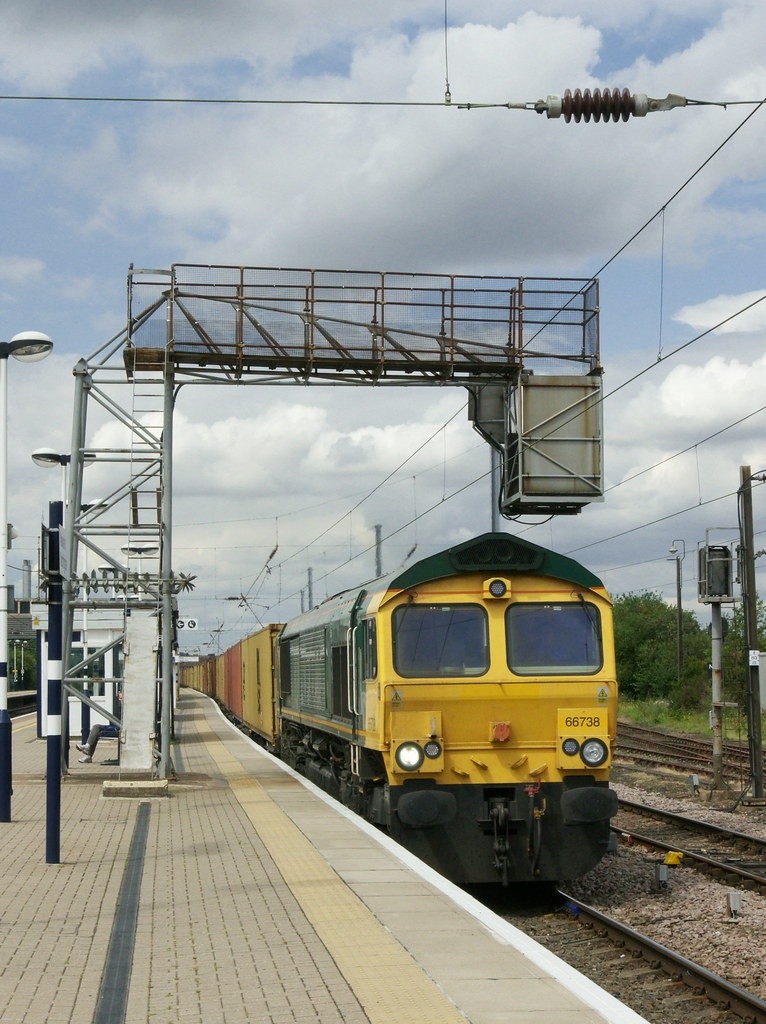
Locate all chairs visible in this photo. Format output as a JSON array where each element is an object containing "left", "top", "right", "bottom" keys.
[{"left": 99, "top": 737, "right": 121, "bottom": 765}]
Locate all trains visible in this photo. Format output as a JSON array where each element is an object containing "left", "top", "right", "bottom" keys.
[{"left": 179, "top": 532, "right": 618, "bottom": 886}]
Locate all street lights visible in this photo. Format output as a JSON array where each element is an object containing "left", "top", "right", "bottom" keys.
[
  {"left": 31, "top": 447, "right": 96, "bottom": 529},
  {"left": 61, "top": 499, "right": 110, "bottom": 745},
  {"left": 669, "top": 539, "right": 685, "bottom": 682},
  {"left": 0, "top": 330, "right": 54, "bottom": 822},
  {"left": 121, "top": 543, "right": 159, "bottom": 601}
]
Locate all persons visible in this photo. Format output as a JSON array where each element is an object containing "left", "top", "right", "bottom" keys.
[{"left": 76, "top": 692, "right": 122, "bottom": 763}]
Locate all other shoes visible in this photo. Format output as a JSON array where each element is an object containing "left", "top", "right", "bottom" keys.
[
  {"left": 76, "top": 744, "right": 90, "bottom": 755},
  {"left": 78, "top": 755, "right": 92, "bottom": 763}
]
